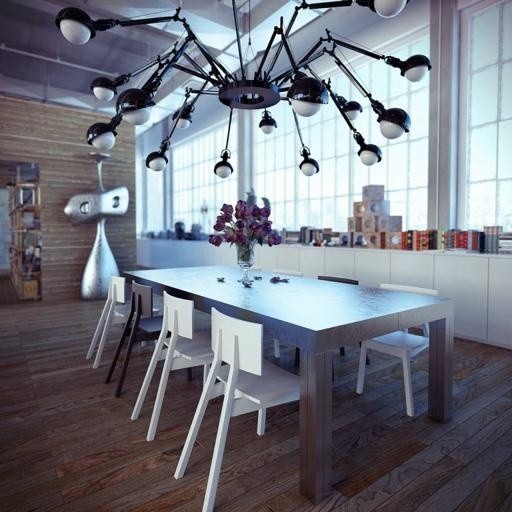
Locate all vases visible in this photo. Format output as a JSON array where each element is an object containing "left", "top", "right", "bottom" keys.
[{"left": 236, "top": 242, "right": 256, "bottom": 285}]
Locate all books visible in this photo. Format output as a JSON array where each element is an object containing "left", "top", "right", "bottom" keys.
[
  {"left": 299, "top": 227, "right": 332, "bottom": 245},
  {"left": 402, "top": 226, "right": 512, "bottom": 255}
]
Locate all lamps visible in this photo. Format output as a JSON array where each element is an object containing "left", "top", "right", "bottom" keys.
[{"left": 54, "top": 0, "right": 438, "bottom": 178}]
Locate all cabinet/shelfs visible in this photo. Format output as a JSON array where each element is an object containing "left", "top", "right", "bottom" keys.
[{"left": 6, "top": 182, "right": 42, "bottom": 304}]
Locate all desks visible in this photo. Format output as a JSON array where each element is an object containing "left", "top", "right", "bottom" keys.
[{"left": 123, "top": 266, "right": 454, "bottom": 506}]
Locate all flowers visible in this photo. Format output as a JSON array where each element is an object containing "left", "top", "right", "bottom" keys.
[{"left": 209, "top": 185, "right": 283, "bottom": 249}]
